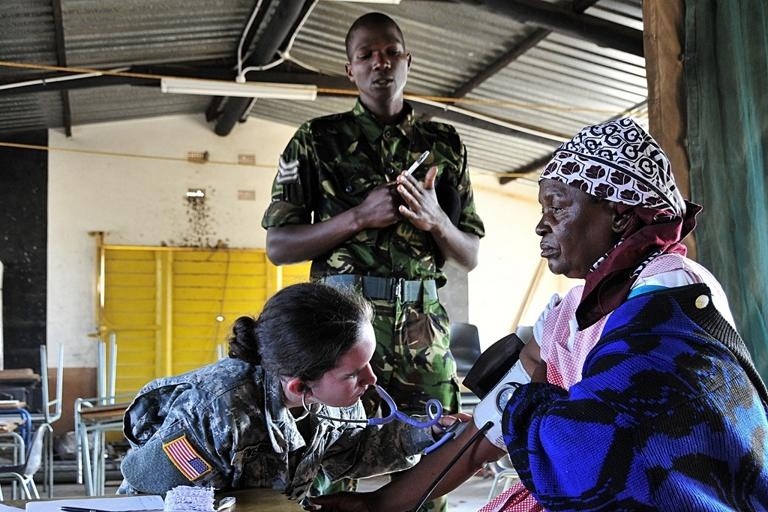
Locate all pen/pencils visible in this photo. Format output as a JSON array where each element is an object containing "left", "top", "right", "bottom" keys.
[
  {"left": 60, "top": 506, "right": 111, "bottom": 512},
  {"left": 408, "top": 151, "right": 429, "bottom": 176}
]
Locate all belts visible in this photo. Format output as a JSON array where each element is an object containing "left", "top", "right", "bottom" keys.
[{"left": 315, "top": 273, "right": 441, "bottom": 306}]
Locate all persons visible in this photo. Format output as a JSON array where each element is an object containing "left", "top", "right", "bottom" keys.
[
  {"left": 259, "top": 12, "right": 486, "bottom": 412},
  {"left": 115, "top": 281, "right": 472, "bottom": 504},
  {"left": 306, "top": 118, "right": 768, "bottom": 512}
]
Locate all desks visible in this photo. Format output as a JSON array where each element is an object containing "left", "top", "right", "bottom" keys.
[{"left": 0, "top": 486, "right": 305, "bottom": 511}]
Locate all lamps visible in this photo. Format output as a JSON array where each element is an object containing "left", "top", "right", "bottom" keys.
[{"left": 159, "top": 74, "right": 318, "bottom": 101}]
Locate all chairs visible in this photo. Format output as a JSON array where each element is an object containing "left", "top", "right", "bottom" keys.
[
  {"left": 449, "top": 322, "right": 482, "bottom": 407},
  {"left": 0, "top": 330, "right": 154, "bottom": 501}
]
